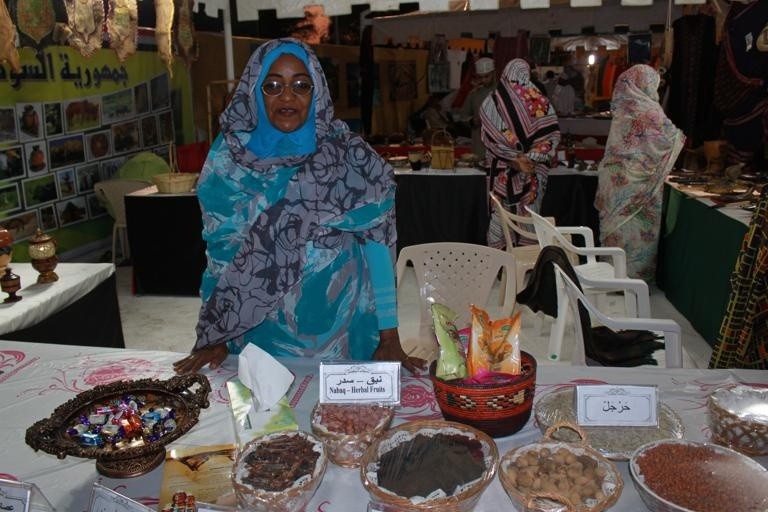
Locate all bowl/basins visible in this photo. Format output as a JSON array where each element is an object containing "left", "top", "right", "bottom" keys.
[
  {"left": 389, "top": 156, "right": 408, "bottom": 168},
  {"left": 629, "top": 436, "right": 768, "bottom": 511}
]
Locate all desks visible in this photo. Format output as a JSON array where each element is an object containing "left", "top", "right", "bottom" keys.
[
  {"left": 0, "top": 338, "right": 767, "bottom": 512},
  {"left": 127, "top": 178, "right": 207, "bottom": 297},
  {"left": 394, "top": 155, "right": 601, "bottom": 245},
  {"left": 656, "top": 171, "right": 768, "bottom": 349},
  {"left": 0, "top": 261, "right": 127, "bottom": 350}
]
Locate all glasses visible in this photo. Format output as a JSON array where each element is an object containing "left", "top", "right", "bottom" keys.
[{"left": 259, "top": 78, "right": 316, "bottom": 98}]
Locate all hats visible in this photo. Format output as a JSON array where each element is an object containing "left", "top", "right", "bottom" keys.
[
  {"left": 558, "top": 71, "right": 569, "bottom": 80},
  {"left": 474, "top": 57, "right": 497, "bottom": 75}
]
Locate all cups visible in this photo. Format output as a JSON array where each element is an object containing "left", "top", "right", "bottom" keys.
[{"left": 407, "top": 150, "right": 423, "bottom": 172}]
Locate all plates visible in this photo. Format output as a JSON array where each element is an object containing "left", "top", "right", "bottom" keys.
[{"left": 534, "top": 382, "right": 685, "bottom": 461}]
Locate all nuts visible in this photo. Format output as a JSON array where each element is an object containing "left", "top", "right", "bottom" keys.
[
  {"left": 632, "top": 443, "right": 768, "bottom": 512},
  {"left": 507, "top": 448, "right": 607, "bottom": 507}
]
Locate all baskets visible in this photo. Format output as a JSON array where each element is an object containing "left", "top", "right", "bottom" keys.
[
  {"left": 358, "top": 417, "right": 500, "bottom": 512},
  {"left": 230, "top": 425, "right": 331, "bottom": 512},
  {"left": 308, "top": 387, "right": 398, "bottom": 474},
  {"left": 497, "top": 420, "right": 627, "bottom": 512},
  {"left": 428, "top": 128, "right": 457, "bottom": 171},
  {"left": 707, "top": 380, "right": 768, "bottom": 457},
  {"left": 424, "top": 343, "right": 541, "bottom": 443},
  {"left": 149, "top": 139, "right": 201, "bottom": 195}
]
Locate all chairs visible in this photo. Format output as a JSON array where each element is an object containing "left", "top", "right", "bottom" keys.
[
  {"left": 484, "top": 192, "right": 578, "bottom": 305},
  {"left": 93, "top": 178, "right": 158, "bottom": 267},
  {"left": 394, "top": 241, "right": 518, "bottom": 361},
  {"left": 523, "top": 205, "right": 652, "bottom": 327},
  {"left": 547, "top": 262, "right": 683, "bottom": 367}
]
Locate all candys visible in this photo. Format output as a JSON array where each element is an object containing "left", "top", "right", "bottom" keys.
[{"left": 68, "top": 394, "right": 177, "bottom": 452}]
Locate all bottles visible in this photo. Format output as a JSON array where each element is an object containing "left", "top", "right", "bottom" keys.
[
  {"left": 28, "top": 228, "right": 59, "bottom": 283},
  {"left": 0, "top": 268, "right": 23, "bottom": 304},
  {"left": 567, "top": 143, "right": 576, "bottom": 169},
  {"left": 564, "top": 127, "right": 573, "bottom": 147}
]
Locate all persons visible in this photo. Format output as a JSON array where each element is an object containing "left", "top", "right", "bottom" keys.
[
  {"left": 532, "top": 65, "right": 597, "bottom": 118},
  {"left": 458, "top": 57, "right": 500, "bottom": 161},
  {"left": 171, "top": 38, "right": 398, "bottom": 379},
  {"left": 478, "top": 58, "right": 561, "bottom": 284},
  {"left": 593, "top": 63, "right": 687, "bottom": 297}
]
